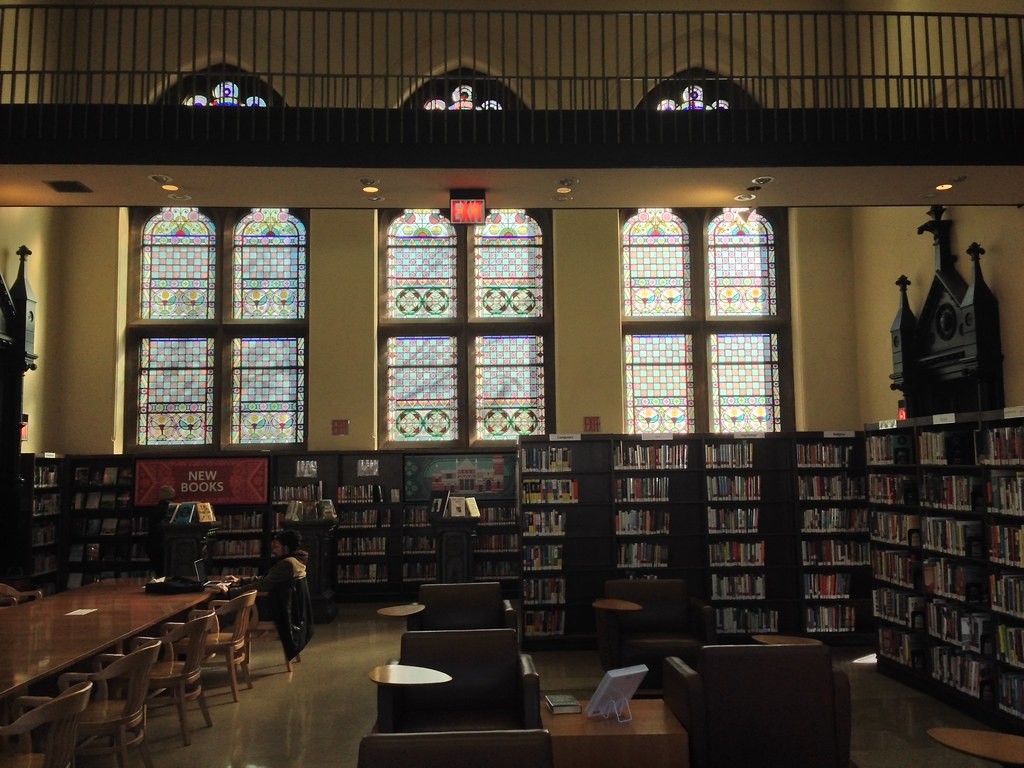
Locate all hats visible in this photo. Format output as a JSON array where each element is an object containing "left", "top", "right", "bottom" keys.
[{"left": 279, "top": 530, "right": 303, "bottom": 551}]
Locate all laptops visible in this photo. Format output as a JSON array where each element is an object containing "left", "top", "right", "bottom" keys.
[{"left": 194, "top": 558, "right": 232, "bottom": 586}]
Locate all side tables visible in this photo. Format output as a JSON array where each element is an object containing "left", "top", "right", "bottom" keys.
[{"left": 537, "top": 698, "right": 689, "bottom": 768}]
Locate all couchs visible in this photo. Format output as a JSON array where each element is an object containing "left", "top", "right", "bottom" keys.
[
  {"left": 357, "top": 728, "right": 554, "bottom": 768},
  {"left": 661, "top": 643, "right": 851, "bottom": 768},
  {"left": 591, "top": 579, "right": 716, "bottom": 692},
  {"left": 406, "top": 582, "right": 519, "bottom": 642},
  {"left": 374, "top": 628, "right": 542, "bottom": 733}
]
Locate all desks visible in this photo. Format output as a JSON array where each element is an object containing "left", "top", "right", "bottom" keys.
[{"left": 0, "top": 574, "right": 246, "bottom": 703}]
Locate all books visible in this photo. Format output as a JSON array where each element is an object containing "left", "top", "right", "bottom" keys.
[
  {"left": 522, "top": 446, "right": 581, "bottom": 636},
  {"left": 982, "top": 424, "right": 1023, "bottom": 722},
  {"left": 795, "top": 443, "right": 868, "bottom": 633},
  {"left": 401, "top": 489, "right": 524, "bottom": 583},
  {"left": 865, "top": 433, "right": 925, "bottom": 671},
  {"left": 611, "top": 440, "right": 691, "bottom": 579},
  {"left": 269, "top": 480, "right": 392, "bottom": 583},
  {"left": 33, "top": 462, "right": 267, "bottom": 598},
  {"left": 704, "top": 439, "right": 783, "bottom": 633},
  {"left": 916, "top": 432, "right": 996, "bottom": 701},
  {"left": 544, "top": 694, "right": 583, "bottom": 715}
]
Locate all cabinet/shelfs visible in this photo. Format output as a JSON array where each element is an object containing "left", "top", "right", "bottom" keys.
[{"left": 0, "top": 406, "right": 1024, "bottom": 740}]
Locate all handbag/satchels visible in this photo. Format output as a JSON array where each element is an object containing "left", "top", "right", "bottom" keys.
[{"left": 146, "top": 576, "right": 205, "bottom": 595}]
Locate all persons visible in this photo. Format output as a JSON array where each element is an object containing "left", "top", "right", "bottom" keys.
[
  {"left": 212, "top": 529, "right": 310, "bottom": 632},
  {"left": 146, "top": 486, "right": 176, "bottom": 578}
]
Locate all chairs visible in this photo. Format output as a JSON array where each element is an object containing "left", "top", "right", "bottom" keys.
[
  {"left": 0, "top": 680, "right": 93, "bottom": 768},
  {"left": 114, "top": 610, "right": 216, "bottom": 747},
  {"left": 15, "top": 639, "right": 162, "bottom": 768},
  {"left": 242, "top": 590, "right": 302, "bottom": 673},
  {"left": 162, "top": 589, "right": 259, "bottom": 703}
]
